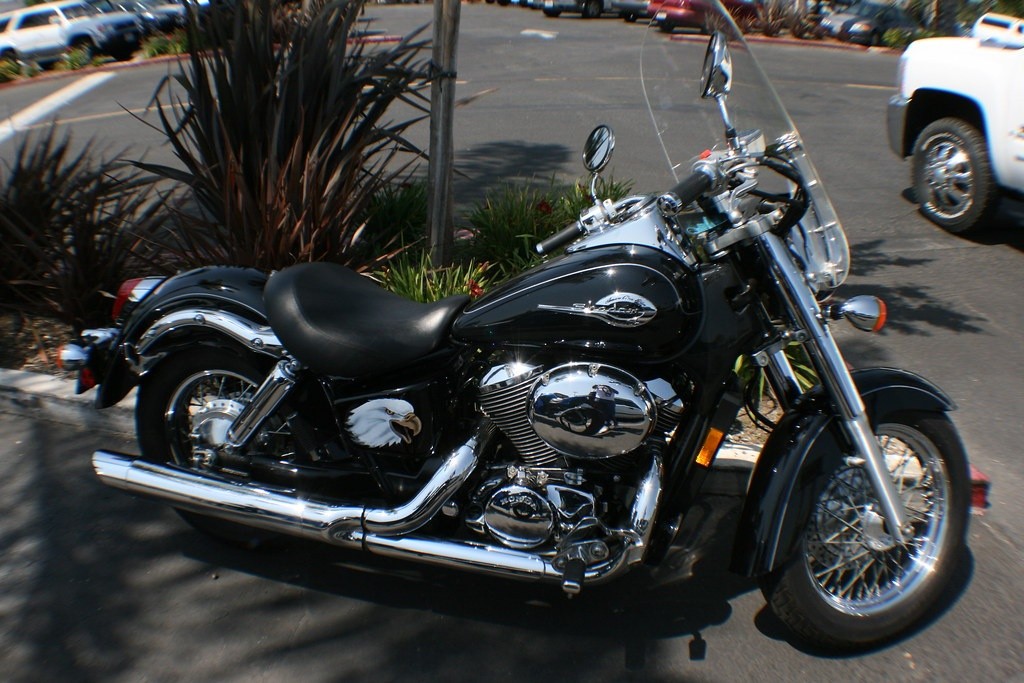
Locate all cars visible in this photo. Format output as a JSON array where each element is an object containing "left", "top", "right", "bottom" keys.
[
  {"left": 803, "top": 1, "right": 921, "bottom": 50},
  {"left": 534, "top": 0, "right": 769, "bottom": 38}
]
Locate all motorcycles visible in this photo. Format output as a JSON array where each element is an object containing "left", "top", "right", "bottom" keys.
[{"left": 50, "top": 0, "right": 973, "bottom": 650}]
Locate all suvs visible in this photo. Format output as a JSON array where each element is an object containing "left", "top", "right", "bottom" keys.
[
  {"left": 0, "top": 0, "right": 212, "bottom": 66},
  {"left": 886, "top": 13, "right": 1023, "bottom": 239}
]
